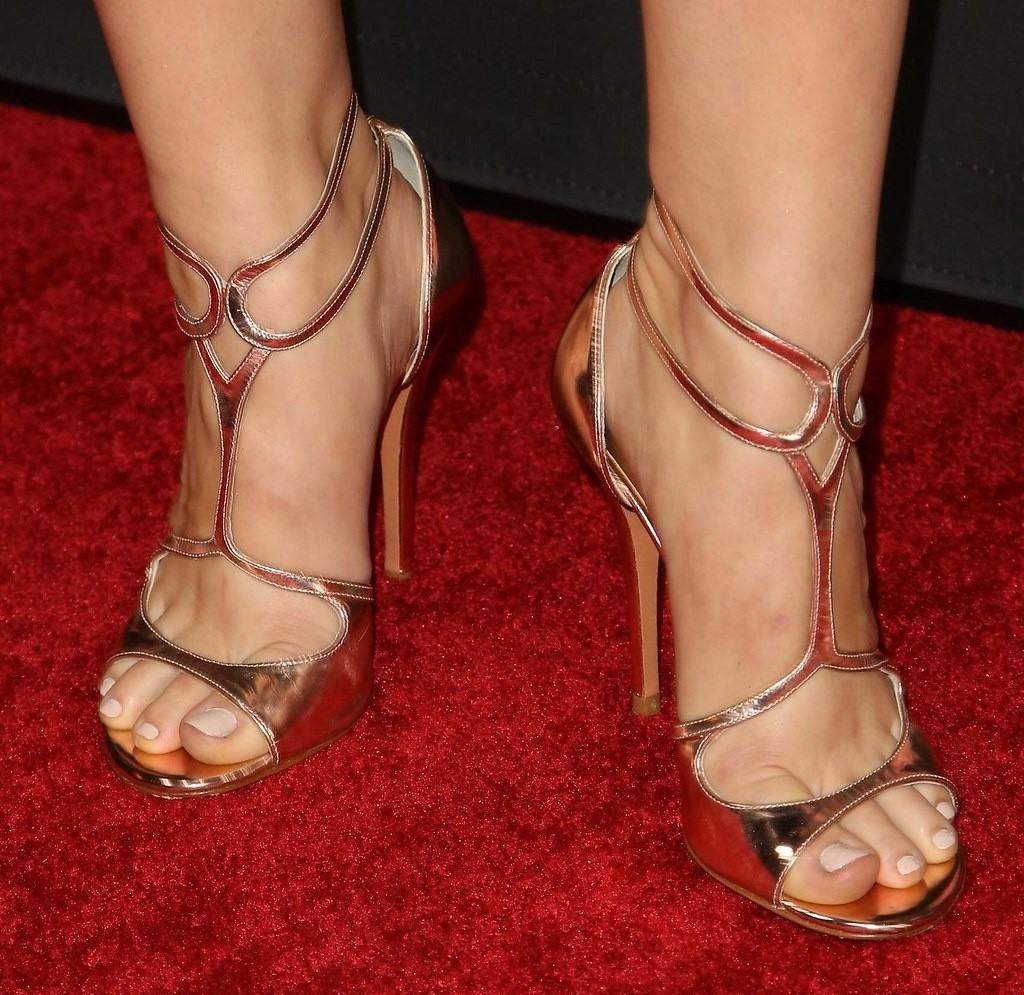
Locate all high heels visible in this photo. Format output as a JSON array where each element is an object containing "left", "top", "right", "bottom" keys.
[
  {"left": 99, "top": 87, "right": 491, "bottom": 801},
  {"left": 549, "top": 191, "right": 968, "bottom": 940}
]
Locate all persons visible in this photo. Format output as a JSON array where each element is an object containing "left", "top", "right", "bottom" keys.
[{"left": 96, "top": 0, "right": 964, "bottom": 942}]
